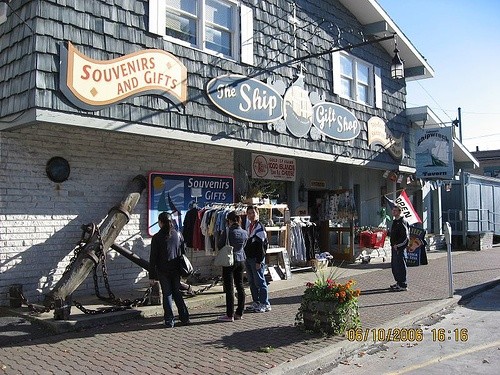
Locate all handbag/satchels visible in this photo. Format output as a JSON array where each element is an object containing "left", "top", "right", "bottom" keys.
[
  {"left": 213, "top": 245, "right": 234, "bottom": 266},
  {"left": 180, "top": 253, "right": 194, "bottom": 277}
]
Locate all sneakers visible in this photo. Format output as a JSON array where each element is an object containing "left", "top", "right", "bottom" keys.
[
  {"left": 246, "top": 300, "right": 261, "bottom": 312},
  {"left": 254, "top": 301, "right": 271, "bottom": 312},
  {"left": 218, "top": 314, "right": 235, "bottom": 321},
  {"left": 234, "top": 312, "right": 242, "bottom": 320}
]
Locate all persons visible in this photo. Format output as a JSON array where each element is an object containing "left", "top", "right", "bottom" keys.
[
  {"left": 390, "top": 206, "right": 410, "bottom": 291},
  {"left": 149, "top": 212, "right": 191, "bottom": 328},
  {"left": 217, "top": 211, "right": 248, "bottom": 321},
  {"left": 244, "top": 205, "right": 271, "bottom": 312}
]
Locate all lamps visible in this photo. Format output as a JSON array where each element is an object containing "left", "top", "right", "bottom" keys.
[{"left": 314, "top": 32, "right": 404, "bottom": 82}]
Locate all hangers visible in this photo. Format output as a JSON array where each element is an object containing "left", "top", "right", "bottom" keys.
[
  {"left": 193, "top": 203, "right": 246, "bottom": 209},
  {"left": 290, "top": 216, "right": 310, "bottom": 224}
]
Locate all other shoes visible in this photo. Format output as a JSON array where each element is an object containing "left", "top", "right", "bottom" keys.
[
  {"left": 390, "top": 282, "right": 407, "bottom": 291},
  {"left": 182, "top": 319, "right": 191, "bottom": 326},
  {"left": 165, "top": 320, "right": 175, "bottom": 328}
]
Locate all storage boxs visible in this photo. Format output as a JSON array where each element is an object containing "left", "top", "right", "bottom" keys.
[
  {"left": 359, "top": 232, "right": 385, "bottom": 248},
  {"left": 243, "top": 198, "right": 260, "bottom": 204}
]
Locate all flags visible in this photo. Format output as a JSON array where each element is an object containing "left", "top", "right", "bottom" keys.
[
  {"left": 384, "top": 190, "right": 422, "bottom": 226},
  {"left": 382, "top": 170, "right": 450, "bottom": 191}
]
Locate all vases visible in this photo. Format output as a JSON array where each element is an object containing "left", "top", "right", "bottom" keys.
[{"left": 304, "top": 299, "right": 352, "bottom": 334}]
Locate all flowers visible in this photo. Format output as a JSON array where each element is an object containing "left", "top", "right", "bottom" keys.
[{"left": 294, "top": 259, "right": 362, "bottom": 334}]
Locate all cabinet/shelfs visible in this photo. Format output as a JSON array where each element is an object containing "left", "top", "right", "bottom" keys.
[{"left": 243, "top": 203, "right": 290, "bottom": 268}]
